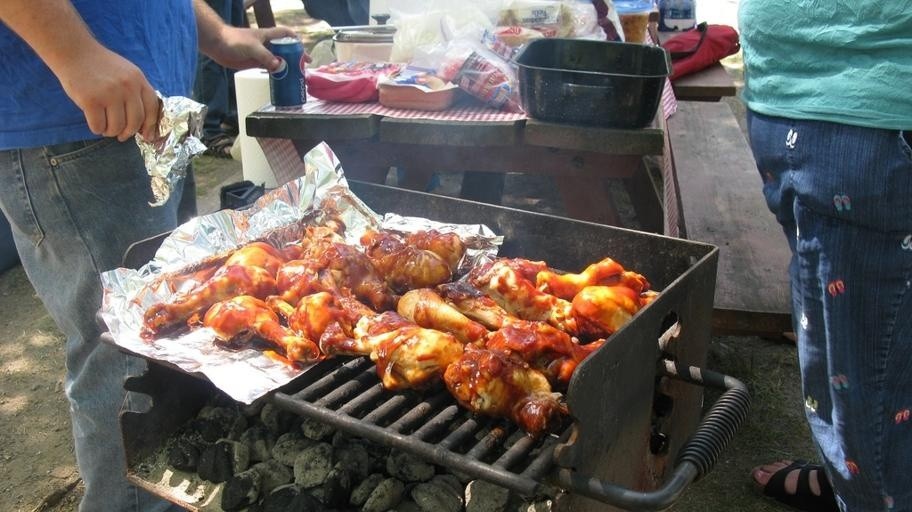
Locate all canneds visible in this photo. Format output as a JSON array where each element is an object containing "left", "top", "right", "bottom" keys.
[{"left": 268, "top": 35, "right": 306, "bottom": 107}]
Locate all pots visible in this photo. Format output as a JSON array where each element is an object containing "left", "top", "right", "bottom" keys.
[{"left": 334, "top": 14, "right": 396, "bottom": 63}]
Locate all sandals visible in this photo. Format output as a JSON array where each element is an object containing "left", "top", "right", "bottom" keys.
[
  {"left": 199, "top": 137, "right": 232, "bottom": 157},
  {"left": 748, "top": 458, "right": 838, "bottom": 509}
]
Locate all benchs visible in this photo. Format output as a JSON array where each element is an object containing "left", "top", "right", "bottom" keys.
[
  {"left": 647, "top": 22, "right": 737, "bottom": 102},
  {"left": 666, "top": 103, "right": 796, "bottom": 341}
]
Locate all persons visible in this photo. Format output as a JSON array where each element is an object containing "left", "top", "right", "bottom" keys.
[
  {"left": 729, "top": 0, "right": 910, "bottom": 509},
  {"left": 1, "top": 0, "right": 315, "bottom": 512},
  {"left": 197, "top": 0, "right": 256, "bottom": 163}
]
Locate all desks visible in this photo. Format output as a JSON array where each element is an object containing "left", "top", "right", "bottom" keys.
[{"left": 246, "top": 93, "right": 664, "bottom": 227}]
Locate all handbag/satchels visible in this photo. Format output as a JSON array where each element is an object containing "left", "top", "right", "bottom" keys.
[{"left": 663, "top": 20, "right": 743, "bottom": 84}]
